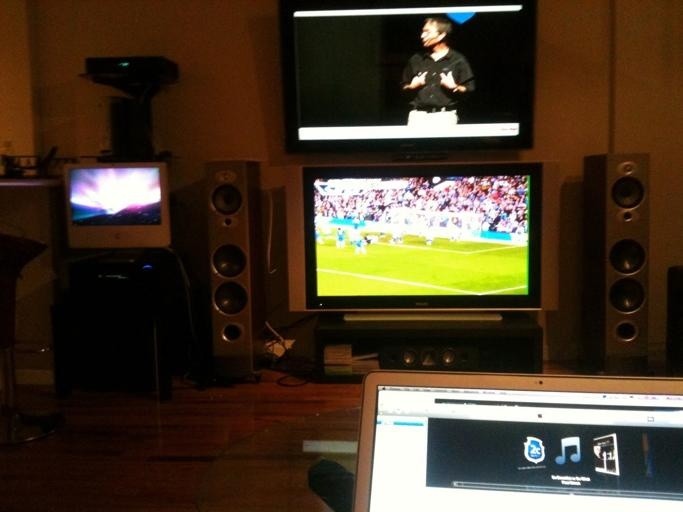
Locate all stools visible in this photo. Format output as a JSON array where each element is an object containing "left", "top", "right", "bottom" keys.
[{"left": 0, "top": 233, "right": 63, "bottom": 446}]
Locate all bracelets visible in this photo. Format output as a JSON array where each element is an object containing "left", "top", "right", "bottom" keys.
[{"left": 452, "top": 85, "right": 461, "bottom": 93}]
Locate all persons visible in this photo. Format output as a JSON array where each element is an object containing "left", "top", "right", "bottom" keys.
[
  {"left": 400, "top": 17, "right": 476, "bottom": 125},
  {"left": 313, "top": 175, "right": 529, "bottom": 257}
]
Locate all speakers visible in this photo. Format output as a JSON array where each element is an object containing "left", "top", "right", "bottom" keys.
[
  {"left": 581, "top": 154, "right": 649, "bottom": 375},
  {"left": 208, "top": 161, "right": 263, "bottom": 383},
  {"left": 395, "top": 343, "right": 462, "bottom": 370}
]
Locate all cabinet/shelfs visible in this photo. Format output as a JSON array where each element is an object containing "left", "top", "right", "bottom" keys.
[{"left": 314, "top": 312, "right": 543, "bottom": 383}]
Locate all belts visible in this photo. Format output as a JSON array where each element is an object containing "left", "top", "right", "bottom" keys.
[{"left": 416, "top": 106, "right": 457, "bottom": 113}]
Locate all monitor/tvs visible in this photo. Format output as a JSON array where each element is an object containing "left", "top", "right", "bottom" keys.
[
  {"left": 284, "top": 161, "right": 561, "bottom": 312},
  {"left": 279, "top": 0, "right": 537, "bottom": 154},
  {"left": 352, "top": 370, "right": 683, "bottom": 512},
  {"left": 64, "top": 162, "right": 171, "bottom": 263}
]
